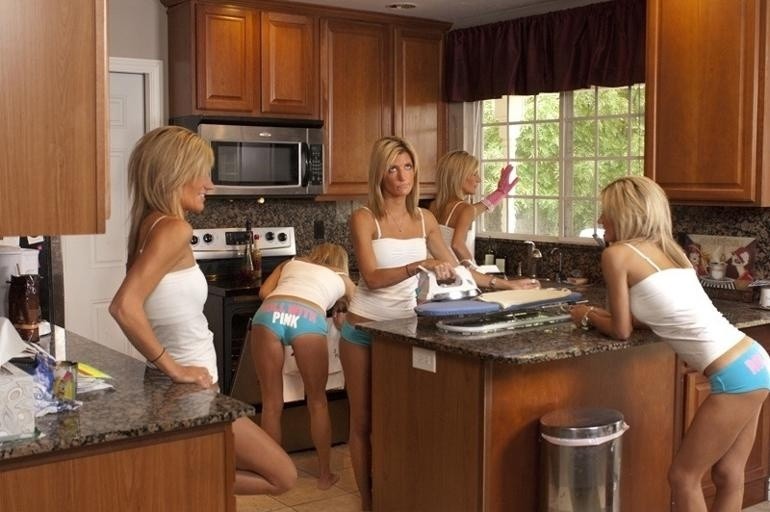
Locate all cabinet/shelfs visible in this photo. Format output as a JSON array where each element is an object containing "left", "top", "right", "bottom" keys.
[
  {"left": 315, "top": 6, "right": 453, "bottom": 203},
  {"left": 643, "top": 0, "right": 769, "bottom": 209},
  {"left": 162, "top": 0, "right": 323, "bottom": 127},
  {"left": 1, "top": 323, "right": 256, "bottom": 511},
  {"left": 354, "top": 302, "right": 769, "bottom": 510},
  {"left": 0, "top": 0, "right": 112, "bottom": 235}
]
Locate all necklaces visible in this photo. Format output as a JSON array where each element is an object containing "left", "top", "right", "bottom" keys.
[{"left": 385, "top": 209, "right": 407, "bottom": 232}]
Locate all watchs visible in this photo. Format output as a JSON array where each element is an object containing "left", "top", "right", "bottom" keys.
[{"left": 580, "top": 307, "right": 594, "bottom": 331}]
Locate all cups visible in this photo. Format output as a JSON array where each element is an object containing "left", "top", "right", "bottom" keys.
[{"left": 704, "top": 262, "right": 728, "bottom": 280}]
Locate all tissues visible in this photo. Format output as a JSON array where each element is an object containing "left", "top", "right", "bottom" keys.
[{"left": 0, "top": 317, "right": 36, "bottom": 443}]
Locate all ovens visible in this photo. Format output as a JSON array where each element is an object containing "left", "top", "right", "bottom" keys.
[{"left": 198, "top": 124, "right": 324, "bottom": 196}]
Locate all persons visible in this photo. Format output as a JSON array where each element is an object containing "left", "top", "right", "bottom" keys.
[
  {"left": 570, "top": 175, "right": 766, "bottom": 512},
  {"left": 338, "top": 137, "right": 539, "bottom": 512},
  {"left": 249, "top": 244, "right": 357, "bottom": 490},
  {"left": 429, "top": 151, "right": 521, "bottom": 271},
  {"left": 110, "top": 126, "right": 297, "bottom": 495}
]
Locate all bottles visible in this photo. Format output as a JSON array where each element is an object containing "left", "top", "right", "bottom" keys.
[
  {"left": 494, "top": 248, "right": 508, "bottom": 275},
  {"left": 483, "top": 244, "right": 495, "bottom": 266}
]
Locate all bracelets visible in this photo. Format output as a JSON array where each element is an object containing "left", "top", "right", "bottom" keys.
[
  {"left": 406, "top": 265, "right": 411, "bottom": 278},
  {"left": 147, "top": 348, "right": 165, "bottom": 364},
  {"left": 489, "top": 277, "right": 497, "bottom": 289}
]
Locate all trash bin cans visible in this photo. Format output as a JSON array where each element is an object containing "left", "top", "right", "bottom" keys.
[{"left": 539, "top": 404, "right": 626, "bottom": 512}]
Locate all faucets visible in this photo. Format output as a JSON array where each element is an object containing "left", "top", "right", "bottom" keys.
[
  {"left": 549, "top": 247, "right": 565, "bottom": 283},
  {"left": 522, "top": 240, "right": 543, "bottom": 278}
]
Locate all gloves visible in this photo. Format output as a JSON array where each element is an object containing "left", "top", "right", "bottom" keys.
[{"left": 478, "top": 164, "right": 521, "bottom": 211}]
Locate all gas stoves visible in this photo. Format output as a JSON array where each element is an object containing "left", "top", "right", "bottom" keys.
[{"left": 205, "top": 267, "right": 264, "bottom": 292}]
